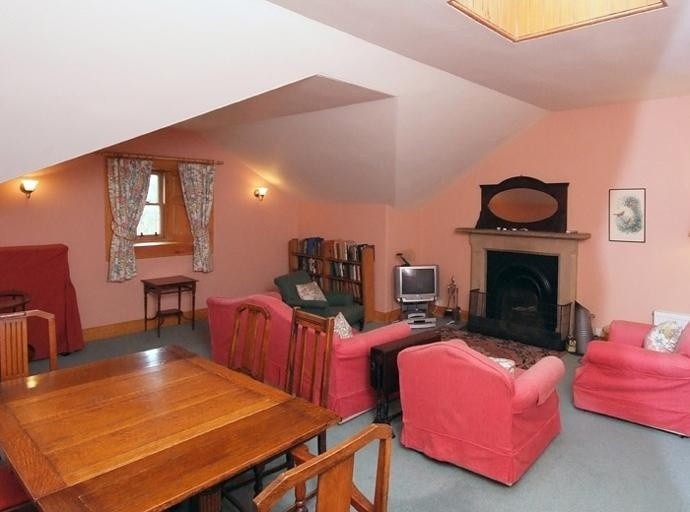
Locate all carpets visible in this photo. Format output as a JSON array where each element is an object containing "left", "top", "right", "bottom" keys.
[{"left": 433, "top": 326, "right": 567, "bottom": 372}]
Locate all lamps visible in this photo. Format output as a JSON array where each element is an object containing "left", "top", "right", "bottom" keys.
[
  {"left": 20, "top": 179, "right": 40, "bottom": 199},
  {"left": 254, "top": 187, "right": 269, "bottom": 201}
]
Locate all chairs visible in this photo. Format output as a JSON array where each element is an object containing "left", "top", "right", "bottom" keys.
[
  {"left": 251, "top": 424, "right": 395, "bottom": 512},
  {"left": 228, "top": 301, "right": 271, "bottom": 383},
  {"left": 0, "top": 309, "right": 58, "bottom": 384},
  {"left": 224, "top": 308, "right": 335, "bottom": 512}
]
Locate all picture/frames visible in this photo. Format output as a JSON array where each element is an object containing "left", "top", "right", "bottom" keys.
[{"left": 608, "top": 187, "right": 646, "bottom": 244}]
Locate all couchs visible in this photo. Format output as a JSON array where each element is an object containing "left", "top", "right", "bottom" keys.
[
  {"left": 571, "top": 318, "right": 690, "bottom": 439},
  {"left": 207, "top": 270, "right": 413, "bottom": 422},
  {"left": 398, "top": 338, "right": 567, "bottom": 487}
]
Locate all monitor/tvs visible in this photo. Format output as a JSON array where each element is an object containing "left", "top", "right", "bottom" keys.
[{"left": 395, "top": 266, "right": 438, "bottom": 300}]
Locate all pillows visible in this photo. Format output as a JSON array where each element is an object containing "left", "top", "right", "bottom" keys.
[
  {"left": 493, "top": 356, "right": 515, "bottom": 374},
  {"left": 642, "top": 321, "right": 682, "bottom": 355},
  {"left": 334, "top": 311, "right": 354, "bottom": 339},
  {"left": 297, "top": 282, "right": 328, "bottom": 303},
  {"left": 274, "top": 269, "right": 329, "bottom": 309}
]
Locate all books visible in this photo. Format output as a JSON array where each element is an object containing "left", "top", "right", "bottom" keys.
[
  {"left": 332, "top": 278, "right": 361, "bottom": 299},
  {"left": 296, "top": 256, "right": 322, "bottom": 274},
  {"left": 329, "top": 241, "right": 368, "bottom": 262},
  {"left": 296, "top": 236, "right": 324, "bottom": 257},
  {"left": 313, "top": 275, "right": 323, "bottom": 289},
  {"left": 331, "top": 261, "right": 362, "bottom": 281}
]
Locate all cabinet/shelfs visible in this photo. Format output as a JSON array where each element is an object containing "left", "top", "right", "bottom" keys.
[{"left": 288, "top": 238, "right": 375, "bottom": 322}]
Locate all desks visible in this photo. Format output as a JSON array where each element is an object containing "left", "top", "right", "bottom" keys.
[
  {"left": 0, "top": 341, "right": 343, "bottom": 512},
  {"left": 140, "top": 276, "right": 200, "bottom": 337}
]
